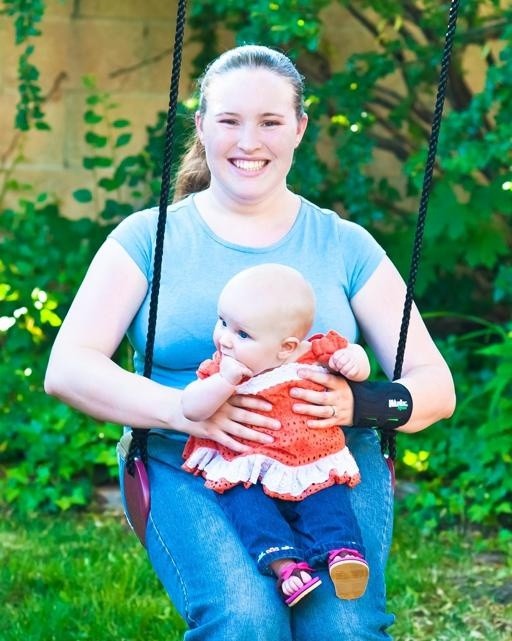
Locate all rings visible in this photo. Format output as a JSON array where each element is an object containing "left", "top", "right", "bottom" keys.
[{"left": 330, "top": 406, "right": 336, "bottom": 417}]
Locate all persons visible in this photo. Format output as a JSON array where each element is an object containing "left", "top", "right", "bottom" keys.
[
  {"left": 42, "top": 42, "right": 457, "bottom": 641},
  {"left": 180, "top": 262, "right": 371, "bottom": 609}
]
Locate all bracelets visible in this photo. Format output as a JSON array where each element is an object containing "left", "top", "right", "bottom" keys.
[{"left": 346, "top": 378, "right": 413, "bottom": 432}]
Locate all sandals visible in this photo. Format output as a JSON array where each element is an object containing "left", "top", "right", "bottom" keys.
[
  {"left": 328, "top": 548, "right": 369, "bottom": 600},
  {"left": 278, "top": 562, "right": 322, "bottom": 608}
]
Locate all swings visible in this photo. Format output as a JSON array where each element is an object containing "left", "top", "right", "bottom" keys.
[{"left": 123, "top": 0, "right": 460, "bottom": 544}]
